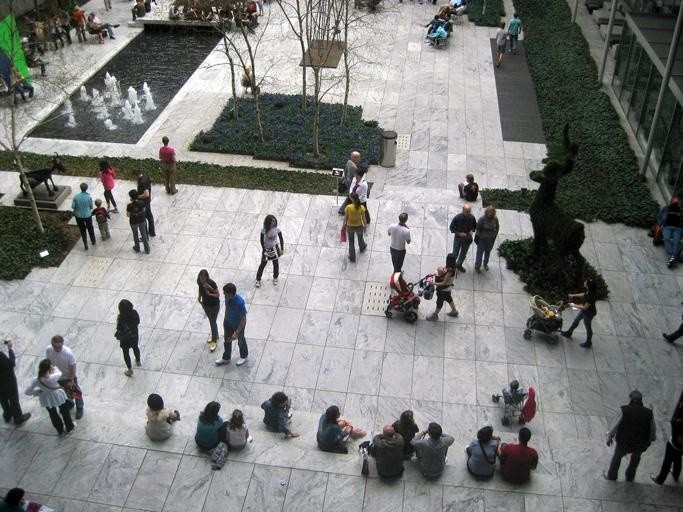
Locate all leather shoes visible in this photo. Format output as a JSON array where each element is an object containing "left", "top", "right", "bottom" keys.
[{"left": 13, "top": 413, "right": 31, "bottom": 424}]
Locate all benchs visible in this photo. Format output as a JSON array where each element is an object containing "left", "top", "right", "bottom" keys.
[
  {"left": 431, "top": 3, "right": 463, "bottom": 50},
  {"left": 0, "top": 8, "right": 110, "bottom": 100}
]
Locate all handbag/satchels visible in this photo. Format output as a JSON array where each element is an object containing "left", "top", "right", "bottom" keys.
[
  {"left": 209, "top": 443, "right": 228, "bottom": 471},
  {"left": 65, "top": 398, "right": 74, "bottom": 410},
  {"left": 341, "top": 226, "right": 347, "bottom": 242},
  {"left": 425, "top": 276, "right": 436, "bottom": 299},
  {"left": 121, "top": 323, "right": 135, "bottom": 344},
  {"left": 264, "top": 244, "right": 282, "bottom": 261},
  {"left": 339, "top": 178, "right": 347, "bottom": 193},
  {"left": 360, "top": 445, "right": 371, "bottom": 475}
]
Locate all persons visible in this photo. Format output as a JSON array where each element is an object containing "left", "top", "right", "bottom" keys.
[
  {"left": 386, "top": 212, "right": 411, "bottom": 274},
  {"left": 260, "top": 392, "right": 300, "bottom": 437},
  {"left": 196, "top": 269, "right": 219, "bottom": 353},
  {"left": 256, "top": 214, "right": 284, "bottom": 289},
  {"left": 506, "top": 12, "right": 522, "bottom": 57},
  {"left": 338, "top": 151, "right": 360, "bottom": 214},
  {"left": 656, "top": 196, "right": 682, "bottom": 267},
  {"left": 24, "top": 358, "right": 76, "bottom": 434},
  {"left": 342, "top": 193, "right": 368, "bottom": 264},
  {"left": 370, "top": 410, "right": 419, "bottom": 483},
  {"left": 466, "top": 426, "right": 500, "bottom": 481},
  {"left": 421, "top": 0, "right": 468, "bottom": 48},
  {"left": 475, "top": 206, "right": 499, "bottom": 274},
  {"left": 409, "top": 423, "right": 455, "bottom": 479},
  {"left": 90, "top": 199, "right": 110, "bottom": 241},
  {"left": 494, "top": 22, "right": 508, "bottom": 68},
  {"left": 662, "top": 321, "right": 682, "bottom": 345},
  {"left": 45, "top": 334, "right": 82, "bottom": 420},
  {"left": 131, "top": 168, "right": 154, "bottom": 237},
  {"left": 448, "top": 205, "right": 477, "bottom": 272},
  {"left": 0, "top": 1, "right": 264, "bottom": 103},
  {"left": 98, "top": 159, "right": 119, "bottom": 214},
  {"left": 158, "top": 136, "right": 178, "bottom": 194},
  {"left": 126, "top": 188, "right": 149, "bottom": 255},
  {"left": 457, "top": 173, "right": 478, "bottom": 202},
  {"left": 112, "top": 298, "right": 142, "bottom": 378},
  {"left": 213, "top": 283, "right": 250, "bottom": 367},
  {"left": 70, "top": 183, "right": 95, "bottom": 250},
  {"left": 650, "top": 395, "right": 683, "bottom": 483},
  {"left": 0, "top": 486, "right": 53, "bottom": 511},
  {"left": 195, "top": 401, "right": 250, "bottom": 451},
  {"left": 0, "top": 336, "right": 30, "bottom": 425},
  {"left": 316, "top": 406, "right": 366, "bottom": 454},
  {"left": 349, "top": 167, "right": 370, "bottom": 224},
  {"left": 604, "top": 390, "right": 655, "bottom": 483},
  {"left": 561, "top": 275, "right": 597, "bottom": 349},
  {"left": 425, "top": 253, "right": 461, "bottom": 321},
  {"left": 498, "top": 428, "right": 537, "bottom": 485},
  {"left": 143, "top": 393, "right": 179, "bottom": 443},
  {"left": 503, "top": 380, "right": 525, "bottom": 404}
]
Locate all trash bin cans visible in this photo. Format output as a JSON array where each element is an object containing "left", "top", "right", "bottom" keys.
[{"left": 380, "top": 131, "right": 398, "bottom": 167}]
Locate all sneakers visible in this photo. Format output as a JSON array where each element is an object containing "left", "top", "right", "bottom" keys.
[
  {"left": 425, "top": 312, "right": 438, "bottom": 320},
  {"left": 650, "top": 473, "right": 657, "bottom": 480},
  {"left": 447, "top": 311, "right": 458, "bottom": 315},
  {"left": 236, "top": 358, "right": 248, "bottom": 365},
  {"left": 209, "top": 342, "right": 217, "bottom": 351},
  {"left": 273, "top": 278, "right": 278, "bottom": 285},
  {"left": 76, "top": 412, "right": 82, "bottom": 419},
  {"left": 215, "top": 358, "right": 231, "bottom": 364},
  {"left": 255, "top": 281, "right": 260, "bottom": 287},
  {"left": 66, "top": 421, "right": 77, "bottom": 433}
]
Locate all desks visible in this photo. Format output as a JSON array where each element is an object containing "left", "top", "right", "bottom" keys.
[{"left": 585, "top": 0, "right": 643, "bottom": 61}]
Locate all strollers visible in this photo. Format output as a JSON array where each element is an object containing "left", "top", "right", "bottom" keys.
[
  {"left": 383, "top": 271, "right": 436, "bottom": 324},
  {"left": 523, "top": 293, "right": 570, "bottom": 345},
  {"left": 492, "top": 380, "right": 536, "bottom": 427}
]
